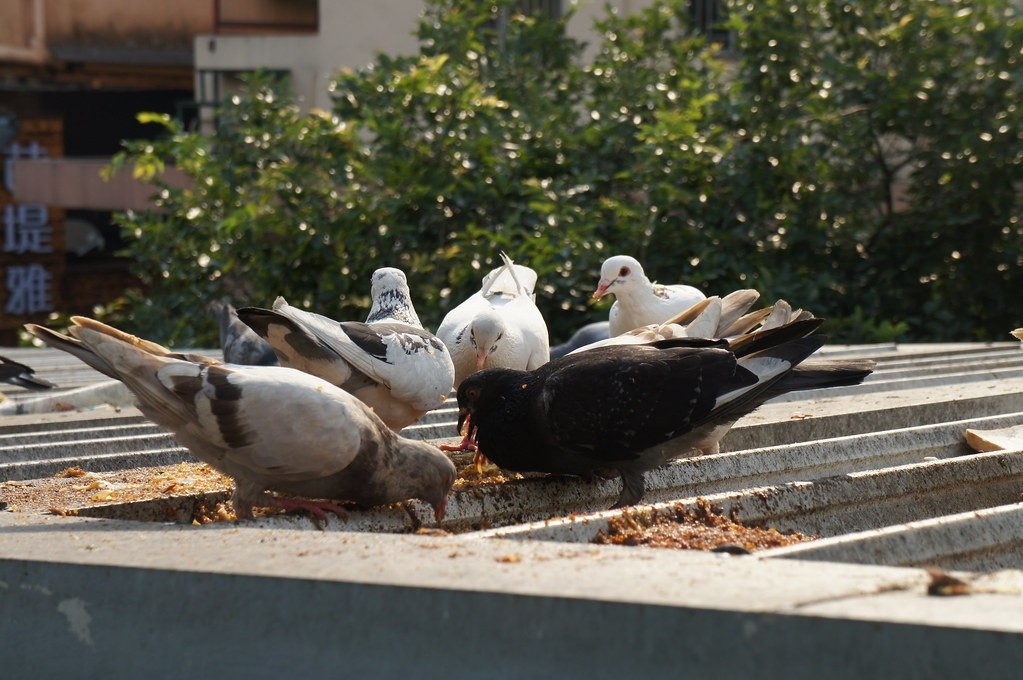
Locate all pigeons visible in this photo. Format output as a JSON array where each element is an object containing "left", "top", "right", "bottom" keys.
[{"left": 22, "top": 251, "right": 876, "bottom": 529}]
